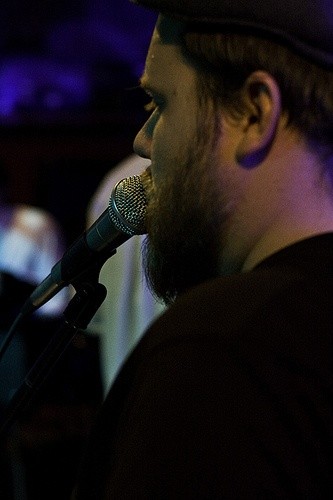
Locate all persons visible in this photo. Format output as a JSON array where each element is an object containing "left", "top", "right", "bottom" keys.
[
  {"left": 47, "top": 0, "right": 332, "bottom": 498},
  {"left": 0, "top": 47, "right": 178, "bottom": 402}
]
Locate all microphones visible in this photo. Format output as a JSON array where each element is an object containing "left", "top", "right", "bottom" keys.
[{"left": 27, "top": 174, "right": 149, "bottom": 314}]
